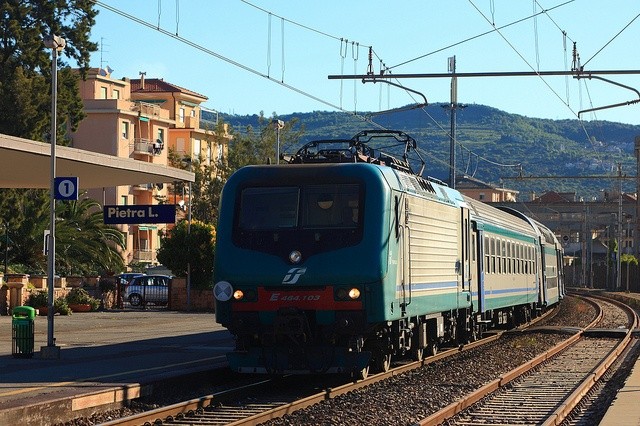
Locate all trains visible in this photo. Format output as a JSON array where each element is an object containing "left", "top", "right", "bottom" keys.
[{"left": 213, "top": 130, "right": 564, "bottom": 380}]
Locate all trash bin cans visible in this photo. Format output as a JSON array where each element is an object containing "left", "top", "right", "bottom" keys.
[{"left": 12, "top": 306, "right": 35, "bottom": 353}]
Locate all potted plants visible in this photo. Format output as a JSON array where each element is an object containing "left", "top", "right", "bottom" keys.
[
  {"left": 28, "top": 290, "right": 74, "bottom": 316},
  {"left": 64, "top": 287, "right": 101, "bottom": 312}
]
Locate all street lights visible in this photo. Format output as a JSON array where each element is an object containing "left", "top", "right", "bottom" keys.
[
  {"left": 41, "top": 35, "right": 66, "bottom": 359},
  {"left": 272, "top": 119, "right": 285, "bottom": 165}
]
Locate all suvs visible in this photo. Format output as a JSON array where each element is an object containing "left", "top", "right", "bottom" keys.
[
  {"left": 119, "top": 273, "right": 167, "bottom": 306},
  {"left": 124, "top": 275, "right": 170, "bottom": 307}
]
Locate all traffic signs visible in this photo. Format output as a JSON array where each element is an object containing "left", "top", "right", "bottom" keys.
[{"left": 55, "top": 177, "right": 77, "bottom": 199}]
